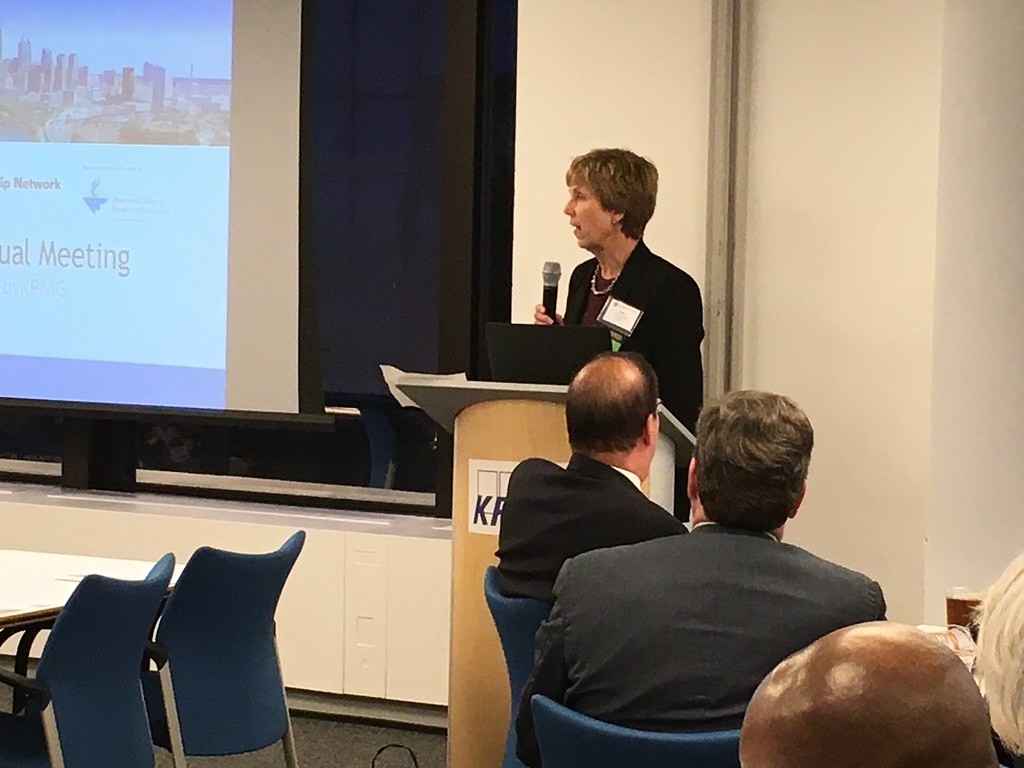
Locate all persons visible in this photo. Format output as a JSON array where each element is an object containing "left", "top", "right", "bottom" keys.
[
  {"left": 976, "top": 553, "right": 1024, "bottom": 768},
  {"left": 536, "top": 148, "right": 703, "bottom": 521},
  {"left": 512, "top": 390, "right": 887, "bottom": 768},
  {"left": 739, "top": 621, "right": 995, "bottom": 767},
  {"left": 494, "top": 352, "right": 689, "bottom": 601}
]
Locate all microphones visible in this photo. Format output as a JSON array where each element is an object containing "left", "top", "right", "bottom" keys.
[{"left": 543, "top": 262, "right": 562, "bottom": 324}]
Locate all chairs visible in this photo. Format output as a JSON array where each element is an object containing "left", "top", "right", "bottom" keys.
[
  {"left": 0, "top": 529, "right": 308, "bottom": 768},
  {"left": 484, "top": 566, "right": 741, "bottom": 768}
]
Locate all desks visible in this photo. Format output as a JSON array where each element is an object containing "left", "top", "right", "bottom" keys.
[{"left": 0, "top": 548, "right": 186, "bottom": 714}]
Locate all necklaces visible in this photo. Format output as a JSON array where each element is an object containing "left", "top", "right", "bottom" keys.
[{"left": 591, "top": 260, "right": 624, "bottom": 296}]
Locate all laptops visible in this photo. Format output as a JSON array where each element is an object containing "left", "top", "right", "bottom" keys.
[{"left": 486, "top": 322, "right": 613, "bottom": 385}]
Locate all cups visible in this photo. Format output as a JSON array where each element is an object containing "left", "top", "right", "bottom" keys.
[{"left": 943, "top": 587, "right": 982, "bottom": 644}]
